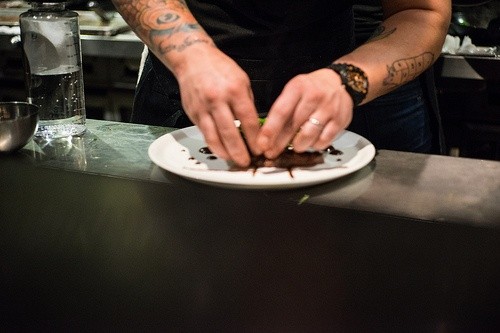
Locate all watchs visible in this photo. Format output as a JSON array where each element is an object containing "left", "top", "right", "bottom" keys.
[{"left": 325, "top": 63, "right": 370, "bottom": 106}]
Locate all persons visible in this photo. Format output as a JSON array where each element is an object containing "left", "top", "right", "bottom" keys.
[{"left": 111, "top": 0, "right": 452, "bottom": 168}]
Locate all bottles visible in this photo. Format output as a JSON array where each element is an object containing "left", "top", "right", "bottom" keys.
[{"left": 19, "top": 0, "right": 87, "bottom": 139}]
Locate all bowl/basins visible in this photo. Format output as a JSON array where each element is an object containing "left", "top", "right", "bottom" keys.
[{"left": 0, "top": 102, "right": 39, "bottom": 155}]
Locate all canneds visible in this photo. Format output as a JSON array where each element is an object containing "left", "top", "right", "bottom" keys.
[{"left": 451, "top": 12, "right": 470, "bottom": 32}]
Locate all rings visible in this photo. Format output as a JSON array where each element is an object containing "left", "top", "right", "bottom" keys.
[{"left": 308, "top": 118, "right": 324, "bottom": 130}]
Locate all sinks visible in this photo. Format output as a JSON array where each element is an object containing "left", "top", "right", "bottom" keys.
[{"left": 0, "top": 8, "right": 131, "bottom": 37}]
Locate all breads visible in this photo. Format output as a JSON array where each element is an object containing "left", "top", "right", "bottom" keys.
[{"left": 243, "top": 148, "right": 323, "bottom": 166}]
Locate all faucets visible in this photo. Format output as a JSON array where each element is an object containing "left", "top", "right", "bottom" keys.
[{"left": 89, "top": 1, "right": 110, "bottom": 23}]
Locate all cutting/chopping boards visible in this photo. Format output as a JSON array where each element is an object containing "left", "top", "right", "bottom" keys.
[{"left": 0, "top": 7, "right": 132, "bottom": 36}]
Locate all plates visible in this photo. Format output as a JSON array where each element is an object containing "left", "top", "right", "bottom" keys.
[{"left": 148, "top": 120, "right": 376, "bottom": 188}]
